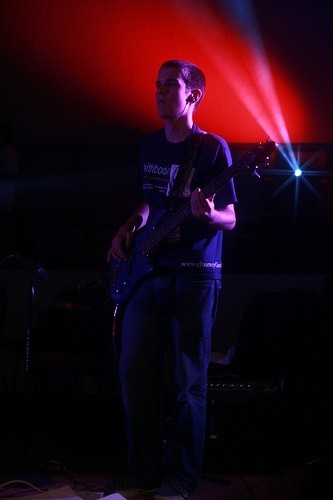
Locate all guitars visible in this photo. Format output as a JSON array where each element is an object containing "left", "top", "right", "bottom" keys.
[{"left": 109, "top": 135, "right": 279, "bottom": 303}]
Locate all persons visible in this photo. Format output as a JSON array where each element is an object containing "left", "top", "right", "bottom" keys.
[{"left": 95, "top": 60, "right": 237, "bottom": 500}]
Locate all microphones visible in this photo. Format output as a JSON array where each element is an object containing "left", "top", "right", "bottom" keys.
[
  {"left": 11, "top": 255, "right": 48, "bottom": 279},
  {"left": 168, "top": 96, "right": 196, "bottom": 118}
]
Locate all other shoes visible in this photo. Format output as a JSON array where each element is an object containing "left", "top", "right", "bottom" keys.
[{"left": 154, "top": 476, "right": 189, "bottom": 500}]
